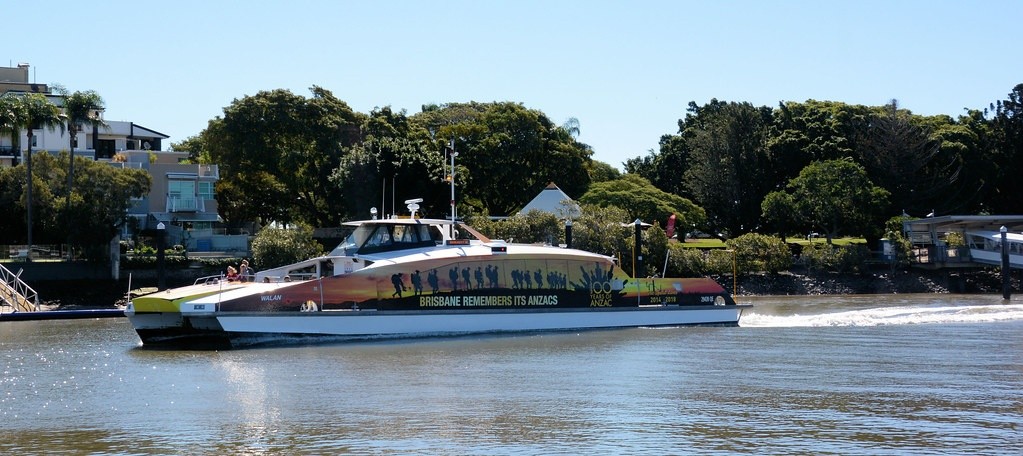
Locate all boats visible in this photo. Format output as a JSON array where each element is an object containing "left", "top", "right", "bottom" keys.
[{"left": 122, "top": 215, "right": 754, "bottom": 350}]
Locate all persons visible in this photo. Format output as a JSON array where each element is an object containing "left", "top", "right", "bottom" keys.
[
  {"left": 390, "top": 264, "right": 566, "bottom": 298},
  {"left": 223, "top": 258, "right": 326, "bottom": 283}
]
